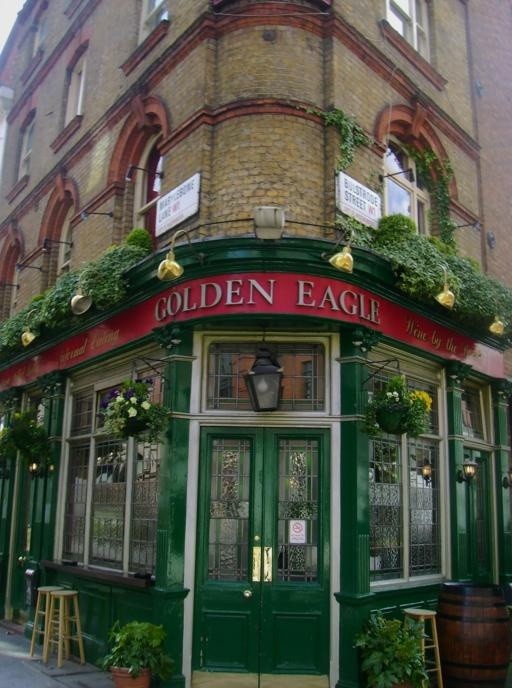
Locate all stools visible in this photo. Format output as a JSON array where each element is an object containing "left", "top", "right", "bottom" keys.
[
  {"left": 400, "top": 606, "right": 445, "bottom": 688},
  {"left": 29, "top": 583, "right": 86, "bottom": 669}
]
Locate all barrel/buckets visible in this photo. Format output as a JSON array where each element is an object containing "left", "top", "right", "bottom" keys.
[{"left": 439, "top": 583, "right": 512, "bottom": 688}]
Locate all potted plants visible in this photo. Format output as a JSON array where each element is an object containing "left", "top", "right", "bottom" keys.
[
  {"left": 95, "top": 618, "right": 176, "bottom": 688},
  {"left": 352, "top": 616, "right": 432, "bottom": 688}
]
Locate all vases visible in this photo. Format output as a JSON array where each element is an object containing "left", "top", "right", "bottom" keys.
[
  {"left": 376, "top": 410, "right": 405, "bottom": 434},
  {"left": 124, "top": 416, "right": 147, "bottom": 433}
]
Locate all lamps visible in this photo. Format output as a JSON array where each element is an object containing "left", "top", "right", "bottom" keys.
[
  {"left": 451, "top": 219, "right": 482, "bottom": 232},
  {"left": 424, "top": 263, "right": 455, "bottom": 311},
  {"left": 81, "top": 210, "right": 115, "bottom": 220},
  {"left": 21, "top": 307, "right": 40, "bottom": 348},
  {"left": 124, "top": 164, "right": 164, "bottom": 184},
  {"left": 70, "top": 266, "right": 107, "bottom": 315},
  {"left": 455, "top": 456, "right": 479, "bottom": 486},
  {"left": 41, "top": 238, "right": 74, "bottom": 253},
  {"left": 157, "top": 230, "right": 205, "bottom": 282},
  {"left": 420, "top": 457, "right": 434, "bottom": 486},
  {"left": 378, "top": 167, "right": 415, "bottom": 183},
  {"left": 488, "top": 310, "right": 505, "bottom": 337},
  {"left": 242, "top": 323, "right": 285, "bottom": 411},
  {"left": 326, "top": 228, "right": 357, "bottom": 275}
]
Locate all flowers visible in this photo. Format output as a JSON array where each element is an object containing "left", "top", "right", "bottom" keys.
[
  {"left": 94, "top": 378, "right": 171, "bottom": 441},
  {"left": 365, "top": 374, "right": 434, "bottom": 441}
]
